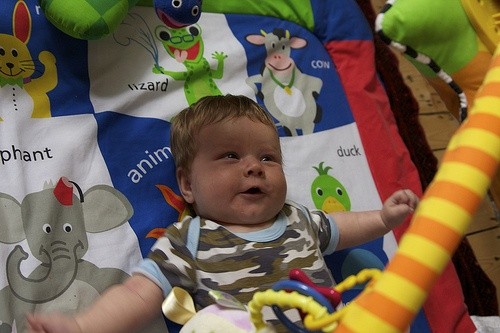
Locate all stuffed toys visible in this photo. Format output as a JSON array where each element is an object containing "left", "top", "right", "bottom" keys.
[
  {"left": 300, "top": 0, "right": 500, "bottom": 333},
  {"left": 43, "top": 0, "right": 203, "bottom": 39}
]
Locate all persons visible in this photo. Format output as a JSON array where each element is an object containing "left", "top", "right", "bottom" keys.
[{"left": 25, "top": 93, "right": 425, "bottom": 333}]
